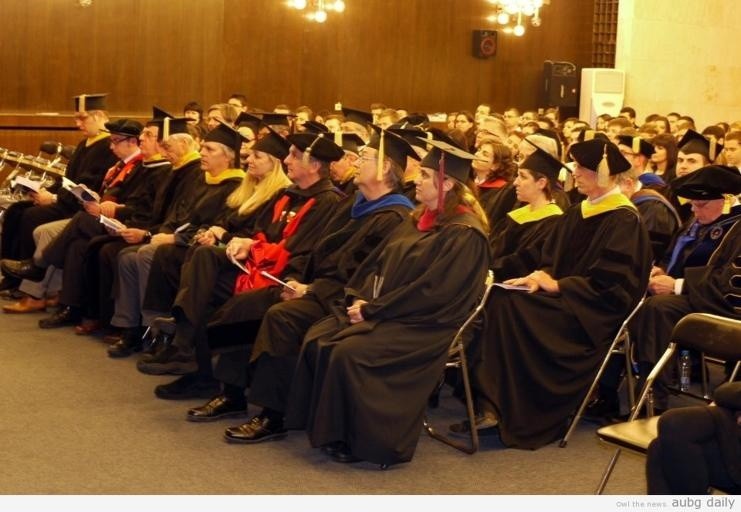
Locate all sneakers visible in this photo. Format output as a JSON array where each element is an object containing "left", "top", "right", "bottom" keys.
[
  {"left": 155, "top": 371, "right": 222, "bottom": 399},
  {"left": 449, "top": 416, "right": 500, "bottom": 436},
  {"left": 137, "top": 345, "right": 200, "bottom": 375}
]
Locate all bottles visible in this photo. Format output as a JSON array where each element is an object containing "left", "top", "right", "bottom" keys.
[{"left": 678, "top": 350, "right": 692, "bottom": 393}]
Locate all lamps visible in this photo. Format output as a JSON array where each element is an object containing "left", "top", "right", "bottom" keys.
[
  {"left": 489, "top": 0, "right": 544, "bottom": 37},
  {"left": 288, "top": 0, "right": 345, "bottom": 24}
]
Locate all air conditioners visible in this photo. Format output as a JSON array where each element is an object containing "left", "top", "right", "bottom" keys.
[{"left": 578, "top": 68, "right": 626, "bottom": 130}]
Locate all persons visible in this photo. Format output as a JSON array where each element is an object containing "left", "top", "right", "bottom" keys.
[{"left": 0, "top": 92, "right": 741, "bottom": 495}]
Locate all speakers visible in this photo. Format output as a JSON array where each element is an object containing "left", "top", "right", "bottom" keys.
[
  {"left": 473, "top": 27, "right": 498, "bottom": 59},
  {"left": 544, "top": 60, "right": 579, "bottom": 109}
]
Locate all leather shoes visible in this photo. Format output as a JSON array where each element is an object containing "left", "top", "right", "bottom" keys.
[
  {"left": 612, "top": 404, "right": 667, "bottom": 422},
  {"left": 39, "top": 306, "right": 83, "bottom": 328},
  {"left": 334, "top": 445, "right": 363, "bottom": 462},
  {"left": 77, "top": 320, "right": 102, "bottom": 335},
  {"left": 322, "top": 443, "right": 342, "bottom": 455},
  {"left": 109, "top": 338, "right": 143, "bottom": 356},
  {"left": 1, "top": 258, "right": 47, "bottom": 282},
  {"left": 224, "top": 414, "right": 289, "bottom": 444},
  {"left": 154, "top": 317, "right": 177, "bottom": 334},
  {"left": 104, "top": 332, "right": 124, "bottom": 344},
  {"left": 4, "top": 295, "right": 47, "bottom": 314},
  {"left": 46, "top": 295, "right": 59, "bottom": 307},
  {"left": 188, "top": 397, "right": 249, "bottom": 420},
  {"left": 583, "top": 398, "right": 621, "bottom": 421}
]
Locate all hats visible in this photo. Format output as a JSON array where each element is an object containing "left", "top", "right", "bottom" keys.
[
  {"left": 570, "top": 139, "right": 632, "bottom": 189},
  {"left": 618, "top": 135, "right": 656, "bottom": 159},
  {"left": 578, "top": 130, "right": 611, "bottom": 143},
  {"left": 205, "top": 118, "right": 250, "bottom": 168},
  {"left": 148, "top": 106, "right": 176, "bottom": 124},
  {"left": 75, "top": 94, "right": 107, "bottom": 112},
  {"left": 428, "top": 128, "right": 465, "bottom": 150},
  {"left": 672, "top": 166, "right": 741, "bottom": 215},
  {"left": 250, "top": 124, "right": 291, "bottom": 160},
  {"left": 678, "top": 129, "right": 725, "bottom": 164},
  {"left": 99, "top": 119, "right": 144, "bottom": 137},
  {"left": 417, "top": 137, "right": 488, "bottom": 215},
  {"left": 399, "top": 112, "right": 427, "bottom": 125},
  {"left": 387, "top": 124, "right": 427, "bottom": 152},
  {"left": 301, "top": 120, "right": 329, "bottom": 134},
  {"left": 342, "top": 108, "right": 373, "bottom": 136},
  {"left": 287, "top": 133, "right": 345, "bottom": 169},
  {"left": 252, "top": 113, "right": 298, "bottom": 129},
  {"left": 518, "top": 137, "right": 572, "bottom": 180},
  {"left": 149, "top": 117, "right": 196, "bottom": 142},
  {"left": 325, "top": 132, "right": 366, "bottom": 157},
  {"left": 367, "top": 122, "right": 422, "bottom": 181},
  {"left": 235, "top": 112, "right": 261, "bottom": 126}
]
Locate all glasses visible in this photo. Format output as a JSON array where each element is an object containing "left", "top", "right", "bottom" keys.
[
  {"left": 109, "top": 137, "right": 130, "bottom": 145},
  {"left": 229, "top": 103, "right": 243, "bottom": 107},
  {"left": 146, "top": 132, "right": 159, "bottom": 139},
  {"left": 520, "top": 116, "right": 535, "bottom": 121},
  {"left": 362, "top": 157, "right": 379, "bottom": 163},
  {"left": 694, "top": 201, "right": 712, "bottom": 209},
  {"left": 74, "top": 116, "right": 89, "bottom": 122}
]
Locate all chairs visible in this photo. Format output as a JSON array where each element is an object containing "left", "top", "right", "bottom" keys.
[
  {"left": 559, "top": 259, "right": 656, "bottom": 448},
  {"left": 1, "top": 141, "right": 78, "bottom": 225},
  {"left": 594, "top": 312, "right": 741, "bottom": 495},
  {"left": 381, "top": 270, "right": 494, "bottom": 471},
  {"left": 634, "top": 196, "right": 681, "bottom": 265}
]
